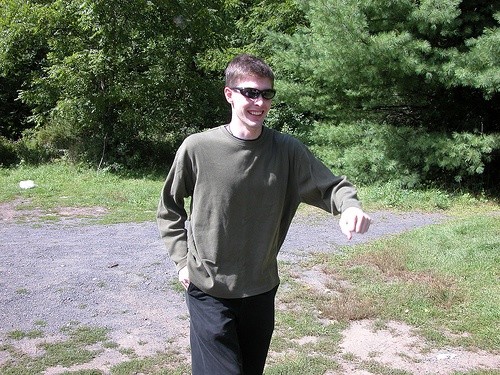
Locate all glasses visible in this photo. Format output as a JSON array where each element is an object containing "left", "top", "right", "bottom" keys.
[{"left": 231, "top": 85, "right": 278, "bottom": 99}]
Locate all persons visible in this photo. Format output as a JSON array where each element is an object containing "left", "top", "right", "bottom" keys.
[{"left": 155, "top": 53, "right": 372, "bottom": 375}]
